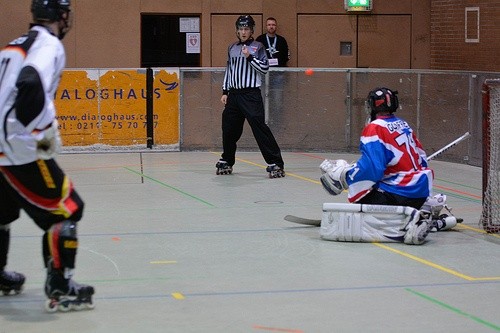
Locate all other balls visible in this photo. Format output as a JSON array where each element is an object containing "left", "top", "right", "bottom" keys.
[{"left": 305, "top": 70, "right": 313, "bottom": 76}]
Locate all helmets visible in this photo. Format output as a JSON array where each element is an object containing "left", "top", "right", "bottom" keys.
[
  {"left": 235, "top": 14, "right": 255, "bottom": 28},
  {"left": 366, "top": 86, "right": 400, "bottom": 120},
  {"left": 31, "top": 0, "right": 74, "bottom": 40}
]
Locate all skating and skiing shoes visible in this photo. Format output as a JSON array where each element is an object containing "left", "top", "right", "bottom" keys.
[
  {"left": 267, "top": 163, "right": 286, "bottom": 178},
  {"left": 216, "top": 159, "right": 232, "bottom": 175},
  {"left": 0, "top": 269, "right": 26, "bottom": 297},
  {"left": 44, "top": 273, "right": 96, "bottom": 313}
]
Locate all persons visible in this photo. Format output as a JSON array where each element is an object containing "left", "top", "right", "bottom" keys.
[
  {"left": 255, "top": 17, "right": 288, "bottom": 129},
  {"left": 0, "top": 0, "right": 94, "bottom": 312},
  {"left": 215, "top": 15, "right": 286, "bottom": 178},
  {"left": 319, "top": 88, "right": 463, "bottom": 243}
]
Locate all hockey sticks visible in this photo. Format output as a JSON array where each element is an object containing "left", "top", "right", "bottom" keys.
[{"left": 284, "top": 130, "right": 473, "bottom": 227}]
